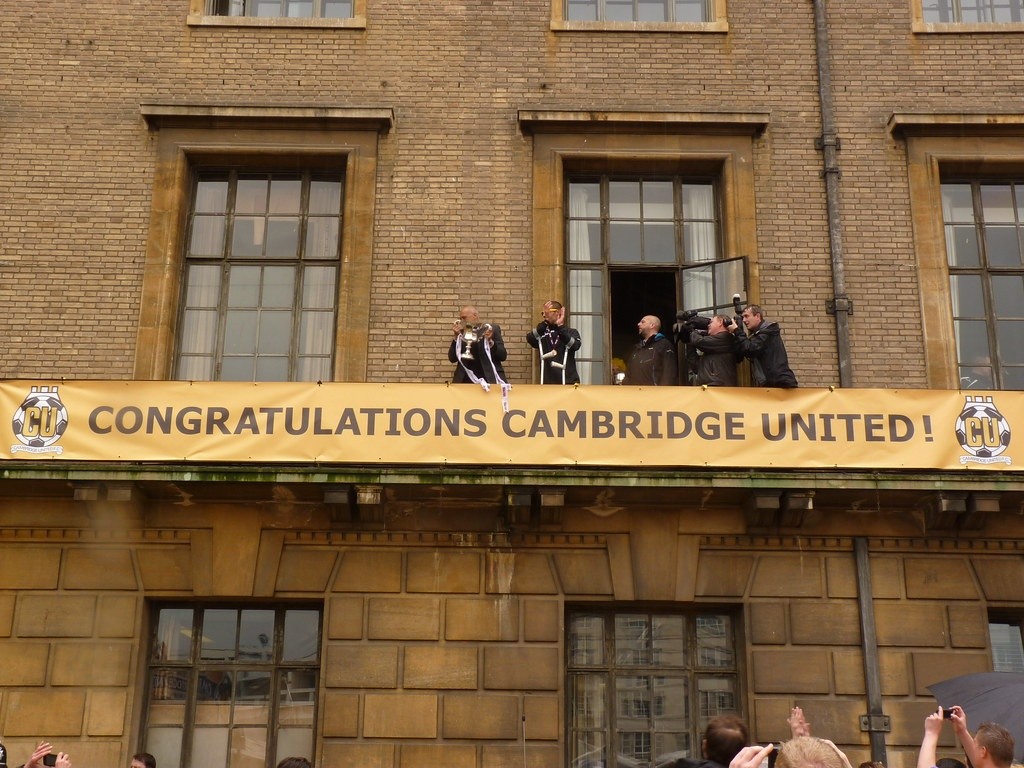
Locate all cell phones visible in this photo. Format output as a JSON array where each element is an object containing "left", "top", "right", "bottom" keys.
[
  {"left": 768, "top": 747, "right": 779, "bottom": 768},
  {"left": 42, "top": 754, "right": 63, "bottom": 767},
  {"left": 936, "top": 709, "right": 955, "bottom": 719}
]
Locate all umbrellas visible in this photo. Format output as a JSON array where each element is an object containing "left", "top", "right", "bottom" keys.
[{"left": 925, "top": 670, "right": 1024, "bottom": 763}]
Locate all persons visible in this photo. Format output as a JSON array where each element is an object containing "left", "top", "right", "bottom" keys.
[
  {"left": 727, "top": 303, "right": 799, "bottom": 388},
  {"left": 0, "top": 740, "right": 72, "bottom": 768},
  {"left": 611, "top": 357, "right": 627, "bottom": 385},
  {"left": 448, "top": 305, "right": 508, "bottom": 384},
  {"left": 277, "top": 757, "right": 311, "bottom": 768},
  {"left": 685, "top": 313, "right": 746, "bottom": 388},
  {"left": 672, "top": 705, "right": 1024, "bottom": 768},
  {"left": 526, "top": 300, "right": 582, "bottom": 384},
  {"left": 130, "top": 752, "right": 156, "bottom": 768},
  {"left": 621, "top": 315, "right": 679, "bottom": 386}
]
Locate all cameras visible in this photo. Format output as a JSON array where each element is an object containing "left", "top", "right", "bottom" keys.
[
  {"left": 679, "top": 310, "right": 711, "bottom": 330},
  {"left": 720, "top": 294, "right": 745, "bottom": 334}
]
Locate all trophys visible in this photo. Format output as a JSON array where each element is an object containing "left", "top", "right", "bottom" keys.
[{"left": 454, "top": 319, "right": 493, "bottom": 360}]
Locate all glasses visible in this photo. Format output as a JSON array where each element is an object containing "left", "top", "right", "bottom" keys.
[{"left": 541, "top": 309, "right": 558, "bottom": 316}]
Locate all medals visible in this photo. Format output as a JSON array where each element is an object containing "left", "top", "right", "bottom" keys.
[{"left": 551, "top": 350, "right": 558, "bottom": 356}]
